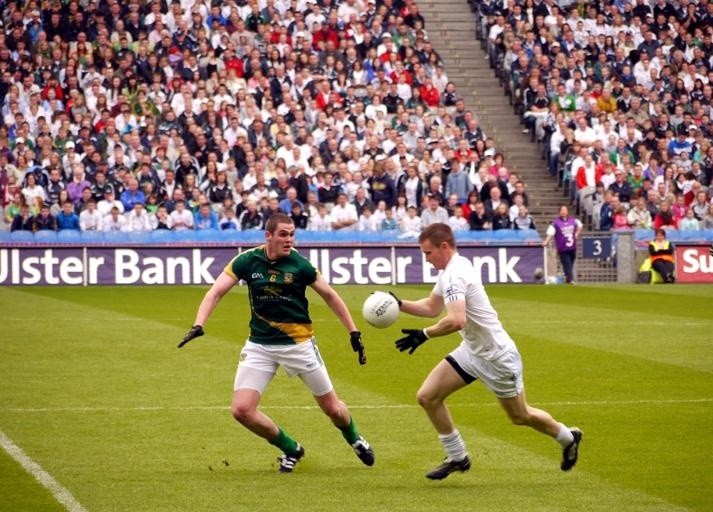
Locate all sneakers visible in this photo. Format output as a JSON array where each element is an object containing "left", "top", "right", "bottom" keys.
[
  {"left": 278, "top": 442, "right": 304, "bottom": 472},
  {"left": 426, "top": 456, "right": 472, "bottom": 480},
  {"left": 348, "top": 435, "right": 375, "bottom": 467},
  {"left": 560, "top": 427, "right": 583, "bottom": 472}
]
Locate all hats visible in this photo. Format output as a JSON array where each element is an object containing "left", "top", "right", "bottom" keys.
[{"left": 0, "top": 0, "right": 713, "bottom": 231}]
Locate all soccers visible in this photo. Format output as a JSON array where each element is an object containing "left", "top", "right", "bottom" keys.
[{"left": 363, "top": 293, "right": 400, "bottom": 329}]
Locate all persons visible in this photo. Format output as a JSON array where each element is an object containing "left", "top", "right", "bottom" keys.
[
  {"left": 0, "top": 0, "right": 713, "bottom": 286},
  {"left": 176, "top": 212, "right": 375, "bottom": 472},
  {"left": 388, "top": 223, "right": 584, "bottom": 481}
]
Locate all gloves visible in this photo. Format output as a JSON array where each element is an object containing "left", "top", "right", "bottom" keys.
[
  {"left": 394, "top": 329, "right": 430, "bottom": 355},
  {"left": 389, "top": 291, "right": 402, "bottom": 307},
  {"left": 349, "top": 331, "right": 367, "bottom": 365},
  {"left": 177, "top": 324, "right": 204, "bottom": 349}
]
